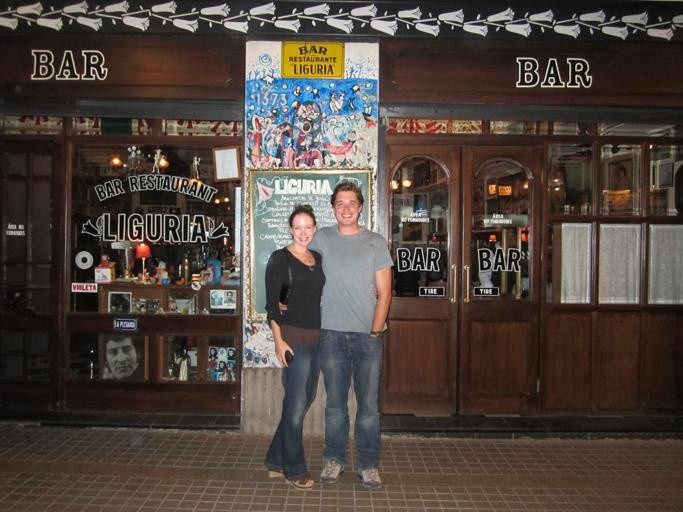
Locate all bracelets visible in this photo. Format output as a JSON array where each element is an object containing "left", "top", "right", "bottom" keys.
[{"left": 370, "top": 331, "right": 381, "bottom": 336}]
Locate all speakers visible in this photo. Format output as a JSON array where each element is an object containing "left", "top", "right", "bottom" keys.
[{"left": 74, "top": 245, "right": 102, "bottom": 312}]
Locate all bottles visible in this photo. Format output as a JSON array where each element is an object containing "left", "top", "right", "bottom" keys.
[{"left": 181, "top": 245, "right": 193, "bottom": 284}]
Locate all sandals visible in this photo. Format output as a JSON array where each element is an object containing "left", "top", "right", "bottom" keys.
[
  {"left": 267, "top": 464, "right": 285, "bottom": 478},
  {"left": 283, "top": 468, "right": 315, "bottom": 491}
]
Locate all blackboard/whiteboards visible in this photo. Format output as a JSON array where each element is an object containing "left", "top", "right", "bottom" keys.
[{"left": 247, "top": 166, "right": 375, "bottom": 324}]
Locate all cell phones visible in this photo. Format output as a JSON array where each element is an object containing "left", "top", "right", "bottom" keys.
[{"left": 285, "top": 351, "right": 293, "bottom": 363}]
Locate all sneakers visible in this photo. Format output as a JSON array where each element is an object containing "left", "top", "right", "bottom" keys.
[
  {"left": 318, "top": 456, "right": 345, "bottom": 484},
  {"left": 356, "top": 464, "right": 383, "bottom": 490}
]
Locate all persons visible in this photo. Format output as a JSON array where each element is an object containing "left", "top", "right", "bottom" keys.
[
  {"left": 101, "top": 335, "right": 143, "bottom": 380},
  {"left": 208, "top": 348, "right": 236, "bottom": 382},
  {"left": 154, "top": 261, "right": 169, "bottom": 285},
  {"left": 474, "top": 226, "right": 532, "bottom": 303},
  {"left": 261, "top": 206, "right": 377, "bottom": 490},
  {"left": 223, "top": 291, "right": 234, "bottom": 302},
  {"left": 275, "top": 182, "right": 394, "bottom": 489}
]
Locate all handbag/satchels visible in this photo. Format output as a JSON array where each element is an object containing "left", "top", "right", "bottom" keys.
[{"left": 275, "top": 247, "right": 295, "bottom": 305}]
